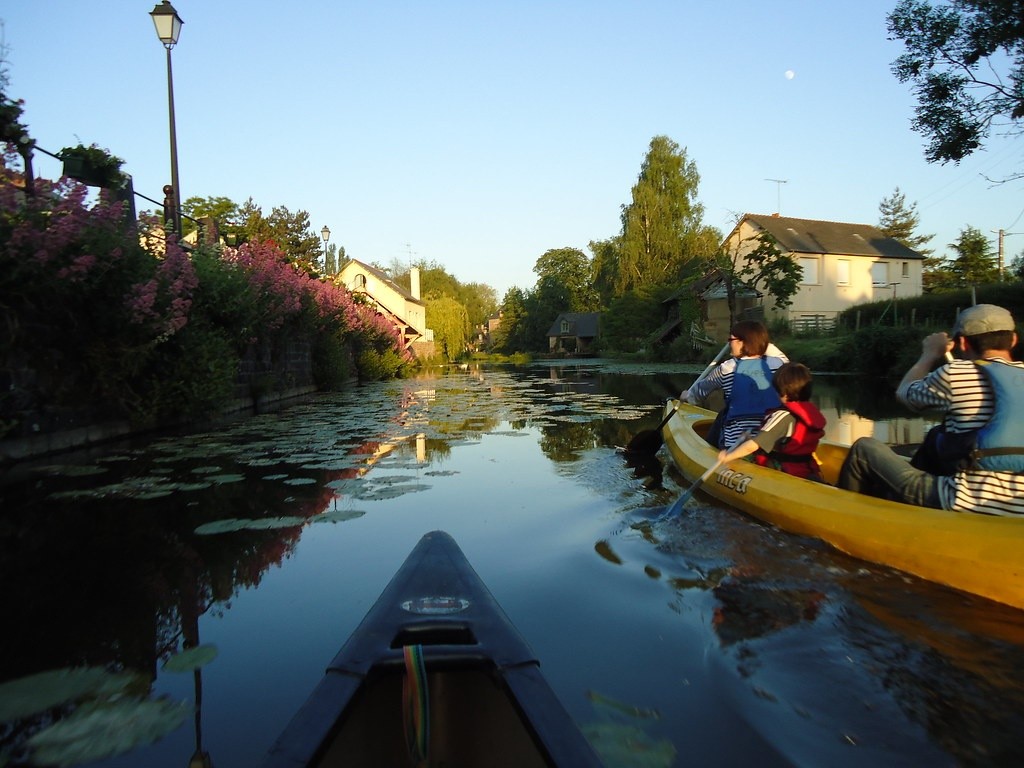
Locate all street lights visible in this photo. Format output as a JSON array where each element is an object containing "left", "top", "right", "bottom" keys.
[
  {"left": 321, "top": 225, "right": 331, "bottom": 275},
  {"left": 149, "top": 0, "right": 184, "bottom": 246}
]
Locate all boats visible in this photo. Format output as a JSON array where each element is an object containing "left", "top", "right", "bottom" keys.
[
  {"left": 263, "top": 531, "right": 603, "bottom": 766},
  {"left": 666, "top": 403, "right": 1024, "bottom": 610}
]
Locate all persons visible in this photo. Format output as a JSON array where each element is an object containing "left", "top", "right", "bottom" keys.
[
  {"left": 681, "top": 319, "right": 791, "bottom": 452},
  {"left": 719, "top": 362, "right": 828, "bottom": 484},
  {"left": 834, "top": 303, "right": 1024, "bottom": 520}
]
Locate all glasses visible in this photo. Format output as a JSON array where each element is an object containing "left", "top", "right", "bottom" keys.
[{"left": 728, "top": 338, "right": 738, "bottom": 342}]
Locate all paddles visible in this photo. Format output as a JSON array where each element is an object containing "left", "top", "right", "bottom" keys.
[
  {"left": 624, "top": 339, "right": 731, "bottom": 462},
  {"left": 661, "top": 425, "right": 756, "bottom": 523}
]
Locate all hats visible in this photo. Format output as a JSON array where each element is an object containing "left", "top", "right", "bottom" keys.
[{"left": 952, "top": 304, "right": 1015, "bottom": 343}]
